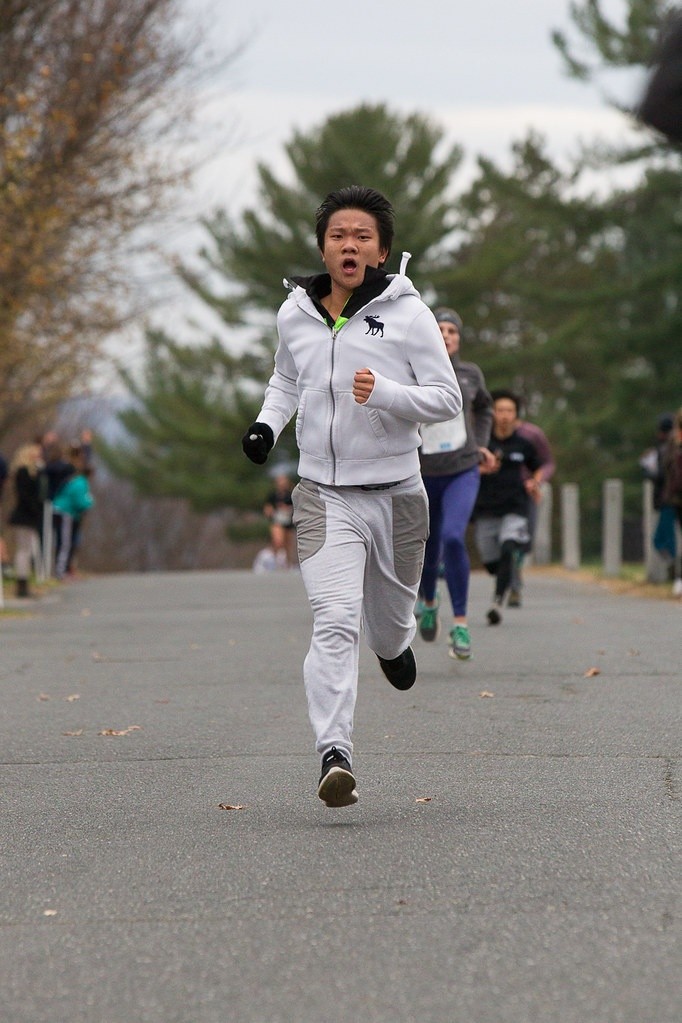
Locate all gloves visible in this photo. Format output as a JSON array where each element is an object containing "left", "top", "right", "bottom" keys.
[{"left": 242, "top": 423, "right": 273, "bottom": 464}]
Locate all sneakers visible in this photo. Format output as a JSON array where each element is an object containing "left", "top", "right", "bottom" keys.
[
  {"left": 317, "top": 749, "right": 359, "bottom": 807},
  {"left": 418, "top": 600, "right": 439, "bottom": 642},
  {"left": 377, "top": 648, "right": 415, "bottom": 691},
  {"left": 446, "top": 624, "right": 471, "bottom": 660}
]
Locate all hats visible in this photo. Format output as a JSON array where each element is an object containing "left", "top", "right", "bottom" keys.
[{"left": 433, "top": 306, "right": 462, "bottom": 331}]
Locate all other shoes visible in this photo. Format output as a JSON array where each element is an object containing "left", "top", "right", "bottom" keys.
[
  {"left": 487, "top": 591, "right": 521, "bottom": 624},
  {"left": 13, "top": 578, "right": 37, "bottom": 598}
]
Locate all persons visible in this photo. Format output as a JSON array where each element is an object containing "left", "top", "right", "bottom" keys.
[
  {"left": 417, "top": 306, "right": 495, "bottom": 663},
  {"left": 0, "top": 429, "right": 97, "bottom": 601},
  {"left": 262, "top": 474, "right": 297, "bottom": 569},
  {"left": 491, "top": 385, "right": 555, "bottom": 609},
  {"left": 470, "top": 393, "right": 545, "bottom": 626},
  {"left": 241, "top": 182, "right": 464, "bottom": 810},
  {"left": 641, "top": 408, "right": 682, "bottom": 596}
]
